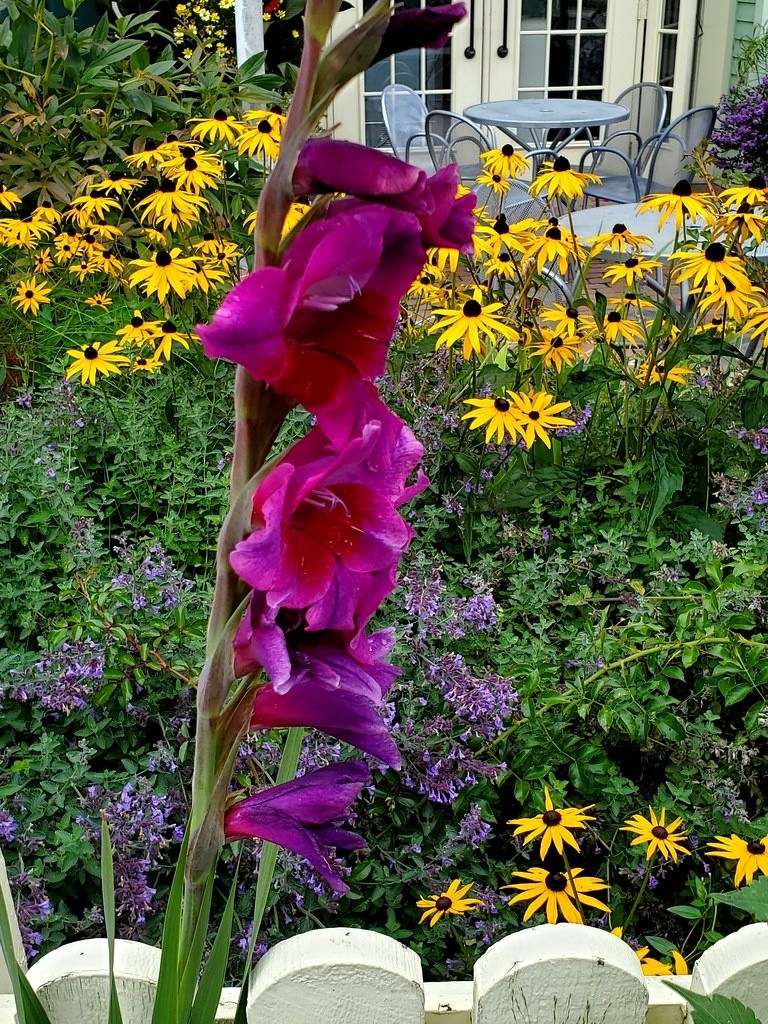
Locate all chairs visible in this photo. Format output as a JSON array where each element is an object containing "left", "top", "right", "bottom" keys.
[{"left": 381, "top": 82, "right": 717, "bottom": 350}]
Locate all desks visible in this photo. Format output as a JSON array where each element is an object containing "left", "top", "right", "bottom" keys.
[
  {"left": 462, "top": 99, "right": 630, "bottom": 223},
  {"left": 556, "top": 202, "right": 768, "bottom": 325}
]
